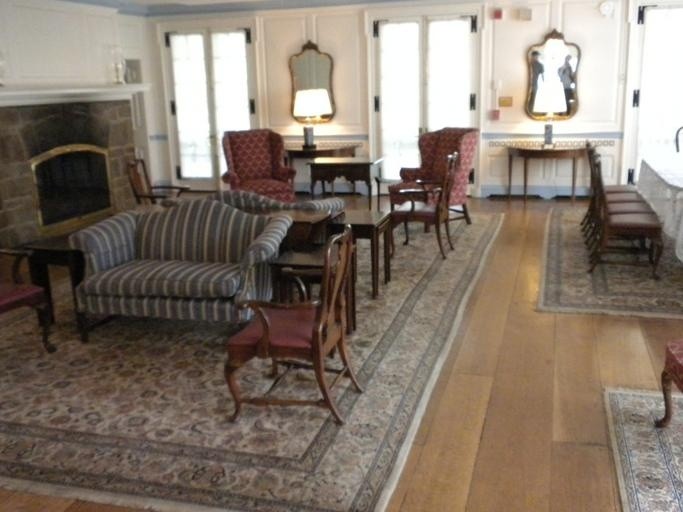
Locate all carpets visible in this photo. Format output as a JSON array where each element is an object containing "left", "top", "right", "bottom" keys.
[
  {"left": 602, "top": 387, "right": 683, "bottom": 512},
  {"left": 534, "top": 206, "right": 682, "bottom": 319},
  {"left": 0, "top": 211, "right": 506, "bottom": 512}
]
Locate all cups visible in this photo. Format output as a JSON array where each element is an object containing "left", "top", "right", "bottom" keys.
[{"left": 303, "top": 126, "right": 313, "bottom": 146}]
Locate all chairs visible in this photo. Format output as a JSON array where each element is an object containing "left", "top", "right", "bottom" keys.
[
  {"left": 224, "top": 222, "right": 364, "bottom": 426},
  {"left": 579, "top": 139, "right": 664, "bottom": 281},
  {"left": 387, "top": 150, "right": 459, "bottom": 261},
  {"left": 222, "top": 128, "right": 297, "bottom": 204},
  {"left": 0, "top": 248, "right": 57, "bottom": 354},
  {"left": 400, "top": 128, "right": 479, "bottom": 233},
  {"left": 127, "top": 157, "right": 191, "bottom": 205}
]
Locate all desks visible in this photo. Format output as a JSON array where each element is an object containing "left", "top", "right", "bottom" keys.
[
  {"left": 306, "top": 156, "right": 383, "bottom": 211},
  {"left": 507, "top": 145, "right": 588, "bottom": 209},
  {"left": 285, "top": 143, "right": 362, "bottom": 197}
]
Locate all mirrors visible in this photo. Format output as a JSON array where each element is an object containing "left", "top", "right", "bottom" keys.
[
  {"left": 525, "top": 28, "right": 581, "bottom": 120},
  {"left": 287, "top": 40, "right": 336, "bottom": 123}
]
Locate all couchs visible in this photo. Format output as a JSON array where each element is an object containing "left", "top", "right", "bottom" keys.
[
  {"left": 68, "top": 198, "right": 294, "bottom": 343},
  {"left": 161, "top": 188, "right": 343, "bottom": 213}
]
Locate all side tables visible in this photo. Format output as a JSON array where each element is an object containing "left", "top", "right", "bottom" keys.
[
  {"left": 268, "top": 242, "right": 354, "bottom": 357},
  {"left": 327, "top": 211, "right": 390, "bottom": 300},
  {"left": 23, "top": 229, "right": 86, "bottom": 329}
]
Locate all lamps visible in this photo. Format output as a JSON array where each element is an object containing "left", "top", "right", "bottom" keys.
[
  {"left": 532, "top": 82, "right": 568, "bottom": 117},
  {"left": 291, "top": 89, "right": 334, "bottom": 121}
]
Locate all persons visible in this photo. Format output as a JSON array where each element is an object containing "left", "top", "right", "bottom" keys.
[
  {"left": 529, "top": 52, "right": 545, "bottom": 114},
  {"left": 558, "top": 55, "right": 576, "bottom": 114}
]
